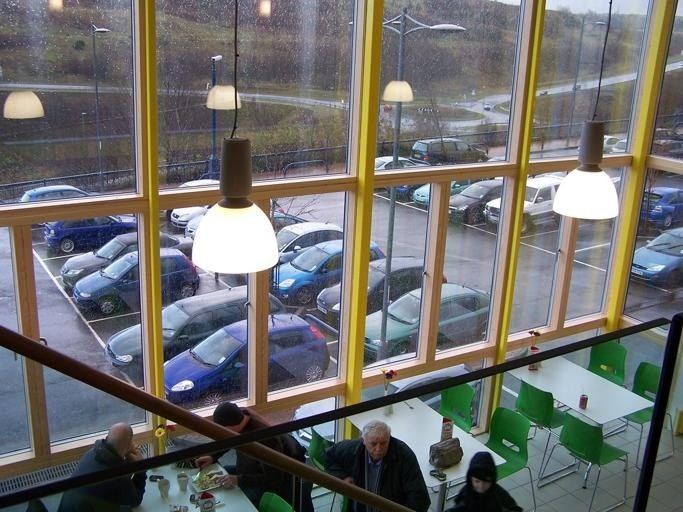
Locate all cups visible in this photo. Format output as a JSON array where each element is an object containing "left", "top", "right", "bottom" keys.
[
  {"left": 157, "top": 478, "right": 170, "bottom": 498},
  {"left": 176, "top": 472, "right": 188, "bottom": 490}
]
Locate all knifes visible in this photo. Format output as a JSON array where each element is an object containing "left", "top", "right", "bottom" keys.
[{"left": 191, "top": 469, "right": 200, "bottom": 482}]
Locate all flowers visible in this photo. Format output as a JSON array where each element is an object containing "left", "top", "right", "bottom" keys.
[
  {"left": 381, "top": 368, "right": 397, "bottom": 390},
  {"left": 528, "top": 331, "right": 540, "bottom": 348}
]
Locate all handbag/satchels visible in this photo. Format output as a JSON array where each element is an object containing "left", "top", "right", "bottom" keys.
[{"left": 429, "top": 437, "right": 463, "bottom": 481}]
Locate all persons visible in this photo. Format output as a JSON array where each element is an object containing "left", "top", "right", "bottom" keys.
[
  {"left": 193, "top": 396, "right": 316, "bottom": 511},
  {"left": 446, "top": 450, "right": 522, "bottom": 511},
  {"left": 320, "top": 418, "right": 433, "bottom": 511},
  {"left": 52, "top": 421, "right": 146, "bottom": 511}
]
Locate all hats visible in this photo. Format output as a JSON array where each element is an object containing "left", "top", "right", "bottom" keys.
[
  {"left": 213, "top": 402, "right": 244, "bottom": 426},
  {"left": 469, "top": 452, "right": 496, "bottom": 483}
]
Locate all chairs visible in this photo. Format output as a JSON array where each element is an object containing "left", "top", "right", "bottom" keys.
[
  {"left": 26, "top": 491, "right": 48, "bottom": 510},
  {"left": 537, "top": 412, "right": 630, "bottom": 511},
  {"left": 282, "top": 436, "right": 315, "bottom": 512},
  {"left": 309, "top": 426, "right": 347, "bottom": 511},
  {"left": 430, "top": 376, "right": 475, "bottom": 494},
  {"left": 445, "top": 407, "right": 537, "bottom": 511},
  {"left": 602, "top": 362, "right": 674, "bottom": 472},
  {"left": 556, "top": 341, "right": 628, "bottom": 436},
  {"left": 513, "top": 378, "right": 577, "bottom": 480},
  {"left": 259, "top": 492, "right": 293, "bottom": 512}
]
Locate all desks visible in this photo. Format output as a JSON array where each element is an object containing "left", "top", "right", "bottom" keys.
[
  {"left": 505, "top": 356, "right": 654, "bottom": 491},
  {"left": 128, "top": 456, "right": 258, "bottom": 512},
  {"left": 344, "top": 396, "right": 507, "bottom": 511}
]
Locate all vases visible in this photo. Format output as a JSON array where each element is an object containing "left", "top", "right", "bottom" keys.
[
  {"left": 528, "top": 350, "right": 540, "bottom": 372},
  {"left": 383, "top": 391, "right": 394, "bottom": 417}
]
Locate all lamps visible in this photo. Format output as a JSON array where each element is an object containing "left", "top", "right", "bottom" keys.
[
  {"left": 3, "top": 90, "right": 45, "bottom": 119},
  {"left": 382, "top": 80, "right": 414, "bottom": 102},
  {"left": 192, "top": 0, "right": 278, "bottom": 275},
  {"left": 206, "top": 62, "right": 242, "bottom": 110},
  {"left": 552, "top": 0, "right": 619, "bottom": 220}
]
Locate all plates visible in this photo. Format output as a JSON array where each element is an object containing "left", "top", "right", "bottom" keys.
[{"left": 189, "top": 471, "right": 224, "bottom": 492}]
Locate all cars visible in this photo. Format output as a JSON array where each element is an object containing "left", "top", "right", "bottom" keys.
[
  {"left": 239, "top": 221, "right": 343, "bottom": 286},
  {"left": 540, "top": 91, "right": 547, "bottom": 96},
  {"left": 61, "top": 230, "right": 193, "bottom": 289},
  {"left": 602, "top": 127, "right": 682, "bottom": 177},
  {"left": 630, "top": 227, "right": 683, "bottom": 290},
  {"left": 572, "top": 85, "right": 581, "bottom": 90},
  {"left": 363, "top": 282, "right": 492, "bottom": 364},
  {"left": 168, "top": 179, "right": 307, "bottom": 241},
  {"left": 484, "top": 104, "right": 490, "bottom": 111},
  {"left": 641, "top": 187, "right": 682, "bottom": 231},
  {"left": 374, "top": 155, "right": 480, "bottom": 207},
  {"left": 448, "top": 179, "right": 503, "bottom": 225}
]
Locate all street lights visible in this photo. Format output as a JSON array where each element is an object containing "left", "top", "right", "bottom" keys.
[
  {"left": 207, "top": 54, "right": 222, "bottom": 174},
  {"left": 346, "top": 10, "right": 464, "bottom": 365},
  {"left": 81, "top": 112, "right": 87, "bottom": 134},
  {"left": 566, "top": 17, "right": 605, "bottom": 151},
  {"left": 89, "top": 18, "right": 112, "bottom": 190}
]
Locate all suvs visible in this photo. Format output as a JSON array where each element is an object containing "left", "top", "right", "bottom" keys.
[
  {"left": 71, "top": 248, "right": 200, "bottom": 317},
  {"left": 103, "top": 284, "right": 288, "bottom": 375},
  {"left": 162, "top": 308, "right": 330, "bottom": 412},
  {"left": 483, "top": 175, "right": 566, "bottom": 235},
  {"left": 20, "top": 185, "right": 101, "bottom": 229},
  {"left": 317, "top": 256, "right": 446, "bottom": 322},
  {"left": 272, "top": 239, "right": 385, "bottom": 305},
  {"left": 43, "top": 215, "right": 138, "bottom": 254},
  {"left": 284, "top": 347, "right": 483, "bottom": 462},
  {"left": 409, "top": 138, "right": 489, "bottom": 166}
]
